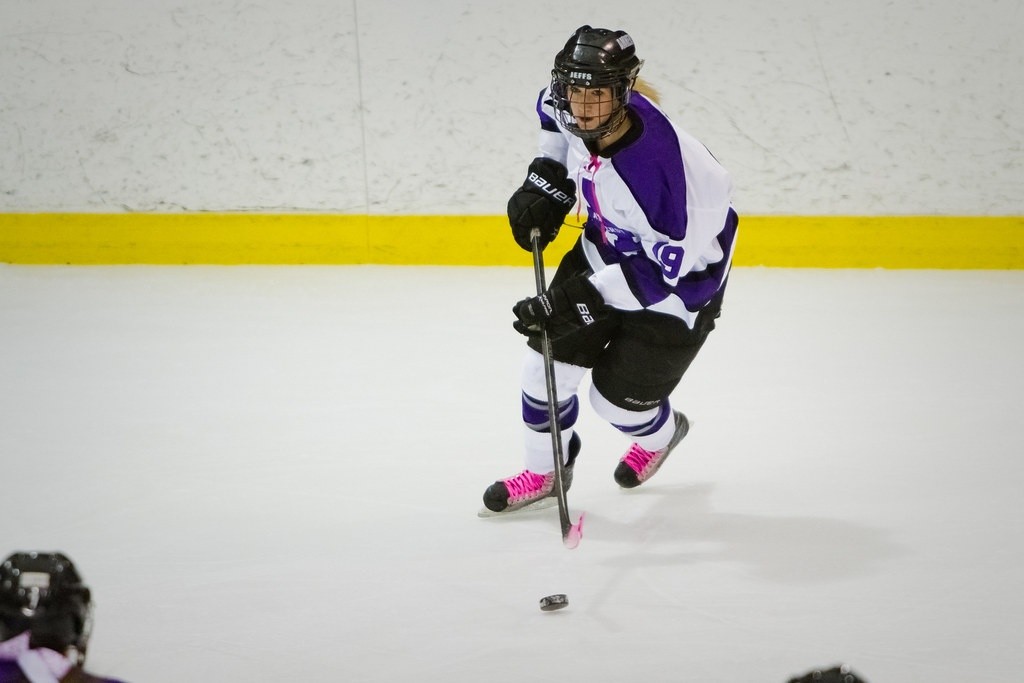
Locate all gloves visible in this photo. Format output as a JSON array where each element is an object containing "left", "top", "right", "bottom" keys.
[
  {"left": 508, "top": 157, "right": 577, "bottom": 251},
  {"left": 513, "top": 270, "right": 608, "bottom": 347}
]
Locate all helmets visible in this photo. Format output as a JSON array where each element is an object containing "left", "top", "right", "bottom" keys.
[
  {"left": 0, "top": 552, "right": 92, "bottom": 656},
  {"left": 549, "top": 25, "right": 644, "bottom": 140}
]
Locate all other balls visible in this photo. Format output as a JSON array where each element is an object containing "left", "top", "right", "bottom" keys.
[{"left": 539, "top": 592, "right": 568, "bottom": 611}]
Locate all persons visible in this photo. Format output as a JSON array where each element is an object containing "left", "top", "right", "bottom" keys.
[
  {"left": 786, "top": 666, "right": 867, "bottom": 683},
  {"left": 478, "top": 24, "right": 739, "bottom": 518},
  {"left": 0, "top": 552, "right": 124, "bottom": 683}
]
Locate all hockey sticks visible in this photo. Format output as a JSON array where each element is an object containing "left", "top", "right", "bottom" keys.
[{"left": 530, "top": 226, "right": 587, "bottom": 548}]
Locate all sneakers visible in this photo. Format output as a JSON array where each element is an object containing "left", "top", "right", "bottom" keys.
[
  {"left": 478, "top": 430, "right": 581, "bottom": 517},
  {"left": 614, "top": 408, "right": 689, "bottom": 488}
]
